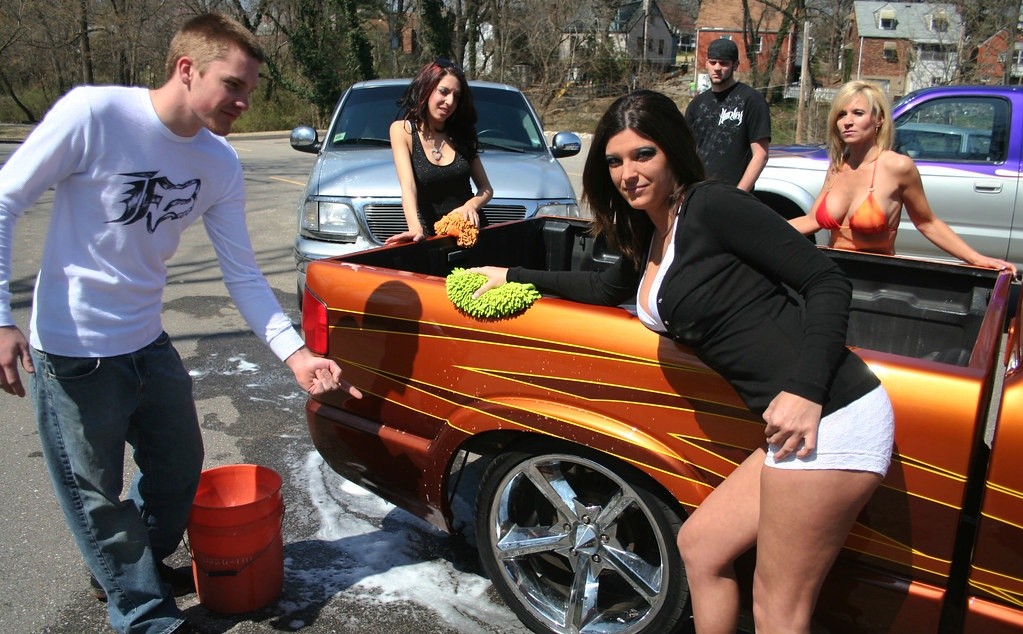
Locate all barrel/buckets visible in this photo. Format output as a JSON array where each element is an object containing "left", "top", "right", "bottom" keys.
[{"left": 181, "top": 465, "right": 286, "bottom": 615}]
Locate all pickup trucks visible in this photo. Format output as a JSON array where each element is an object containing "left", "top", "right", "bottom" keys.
[{"left": 305, "top": 215, "right": 1023, "bottom": 633}]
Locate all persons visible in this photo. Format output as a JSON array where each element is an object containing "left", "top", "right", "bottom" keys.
[
  {"left": 383, "top": 62, "right": 495, "bottom": 246},
  {"left": 465, "top": 91, "right": 895, "bottom": 634},
  {"left": 786, "top": 78, "right": 1018, "bottom": 284},
  {"left": 0, "top": 12, "right": 362, "bottom": 634},
  {"left": 683, "top": 38, "right": 772, "bottom": 196}
]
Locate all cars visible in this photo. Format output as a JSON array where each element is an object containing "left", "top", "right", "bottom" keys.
[
  {"left": 289, "top": 80, "right": 581, "bottom": 312},
  {"left": 750, "top": 86, "right": 1023, "bottom": 269}
]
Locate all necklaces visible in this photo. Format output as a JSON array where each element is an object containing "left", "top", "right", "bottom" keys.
[
  {"left": 416, "top": 121, "right": 451, "bottom": 162},
  {"left": 654, "top": 208, "right": 678, "bottom": 241}
]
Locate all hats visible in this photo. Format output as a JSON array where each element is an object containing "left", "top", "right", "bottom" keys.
[{"left": 708, "top": 39, "right": 739, "bottom": 61}]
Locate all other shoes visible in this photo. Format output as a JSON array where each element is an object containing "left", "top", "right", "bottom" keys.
[{"left": 88, "top": 574, "right": 191, "bottom": 599}]
